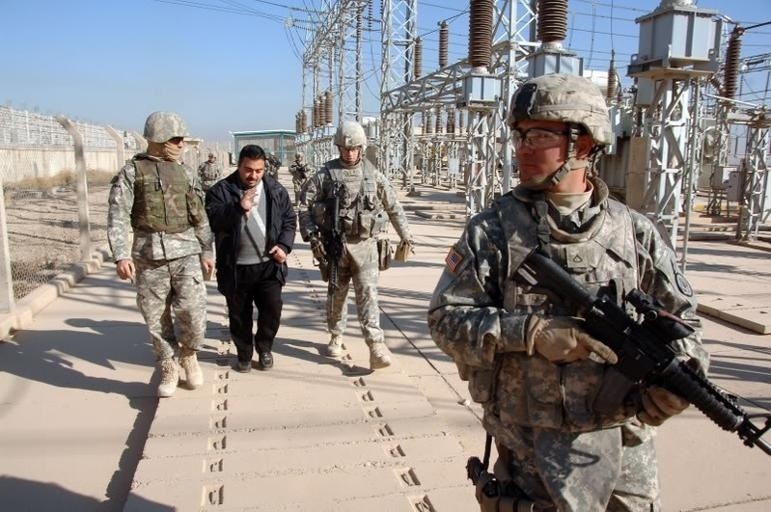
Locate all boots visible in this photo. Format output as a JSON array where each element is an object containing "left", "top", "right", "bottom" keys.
[
  {"left": 369, "top": 342, "right": 391, "bottom": 368},
  {"left": 327, "top": 333, "right": 342, "bottom": 356},
  {"left": 179, "top": 349, "right": 204, "bottom": 389},
  {"left": 157, "top": 357, "right": 179, "bottom": 398}
]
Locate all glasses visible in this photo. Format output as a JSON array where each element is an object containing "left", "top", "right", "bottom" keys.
[{"left": 509, "top": 128, "right": 572, "bottom": 147}]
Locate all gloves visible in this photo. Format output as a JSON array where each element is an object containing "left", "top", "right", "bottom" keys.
[
  {"left": 311, "top": 239, "right": 327, "bottom": 260},
  {"left": 526, "top": 314, "right": 619, "bottom": 365},
  {"left": 400, "top": 235, "right": 416, "bottom": 249},
  {"left": 639, "top": 386, "right": 689, "bottom": 426}
]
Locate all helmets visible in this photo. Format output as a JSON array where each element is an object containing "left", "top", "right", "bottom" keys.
[
  {"left": 208, "top": 153, "right": 217, "bottom": 158},
  {"left": 295, "top": 152, "right": 303, "bottom": 158},
  {"left": 334, "top": 122, "right": 365, "bottom": 147},
  {"left": 508, "top": 73, "right": 612, "bottom": 144},
  {"left": 144, "top": 111, "right": 190, "bottom": 144}
]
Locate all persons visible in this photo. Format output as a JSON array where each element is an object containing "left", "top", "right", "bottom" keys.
[
  {"left": 105, "top": 109, "right": 216, "bottom": 399},
  {"left": 204, "top": 143, "right": 297, "bottom": 373},
  {"left": 196, "top": 150, "right": 312, "bottom": 207},
  {"left": 297, "top": 120, "right": 417, "bottom": 371},
  {"left": 424, "top": 71, "right": 711, "bottom": 512}
]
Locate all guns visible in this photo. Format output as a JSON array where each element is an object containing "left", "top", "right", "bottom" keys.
[
  {"left": 507, "top": 245, "right": 771, "bottom": 458},
  {"left": 295, "top": 159, "right": 307, "bottom": 178},
  {"left": 324, "top": 185, "right": 344, "bottom": 321}
]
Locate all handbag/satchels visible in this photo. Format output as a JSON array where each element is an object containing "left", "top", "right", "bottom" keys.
[{"left": 378, "top": 239, "right": 395, "bottom": 270}]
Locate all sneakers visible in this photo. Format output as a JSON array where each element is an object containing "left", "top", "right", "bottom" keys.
[
  {"left": 238, "top": 361, "right": 251, "bottom": 373},
  {"left": 259, "top": 351, "right": 274, "bottom": 370}
]
